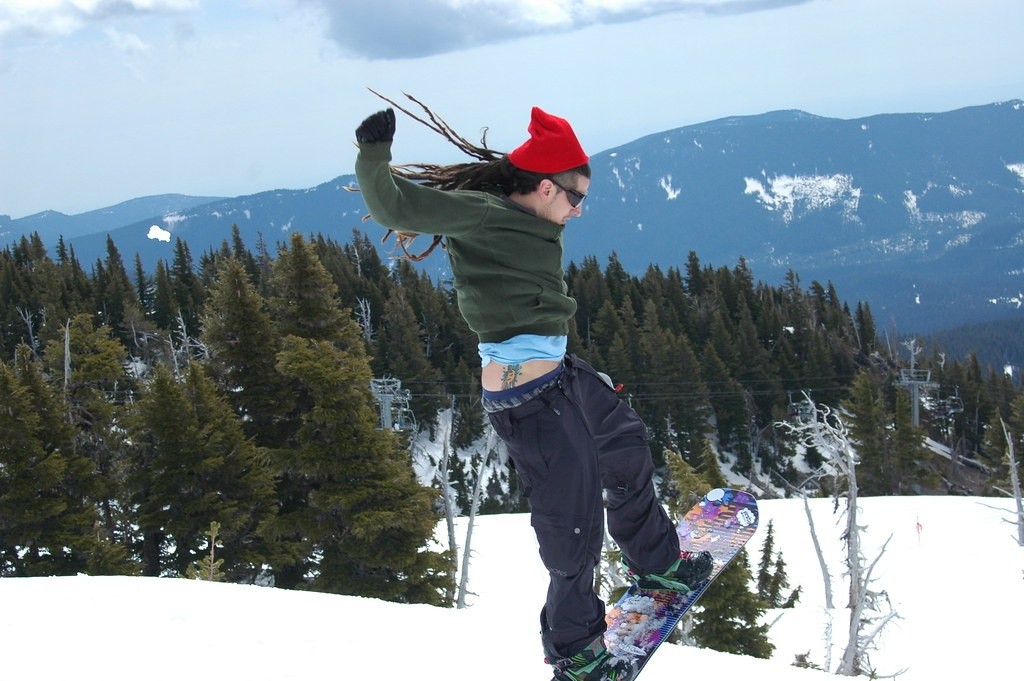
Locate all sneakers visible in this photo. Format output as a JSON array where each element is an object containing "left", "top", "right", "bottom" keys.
[
  {"left": 620, "top": 549, "right": 714, "bottom": 590},
  {"left": 544, "top": 634, "right": 634, "bottom": 681}
]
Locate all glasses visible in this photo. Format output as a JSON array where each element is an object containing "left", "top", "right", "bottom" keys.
[{"left": 547, "top": 176, "right": 587, "bottom": 210}]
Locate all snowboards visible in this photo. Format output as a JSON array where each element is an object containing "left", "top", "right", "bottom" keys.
[{"left": 552, "top": 488, "right": 760, "bottom": 681}]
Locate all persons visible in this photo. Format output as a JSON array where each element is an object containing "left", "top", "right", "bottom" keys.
[{"left": 354, "top": 102, "right": 711, "bottom": 681}]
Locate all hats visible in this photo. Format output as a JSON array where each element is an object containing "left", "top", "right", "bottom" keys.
[{"left": 506, "top": 106, "right": 589, "bottom": 174}]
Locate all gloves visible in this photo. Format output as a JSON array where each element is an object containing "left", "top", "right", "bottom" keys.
[{"left": 355, "top": 108, "right": 396, "bottom": 143}]
